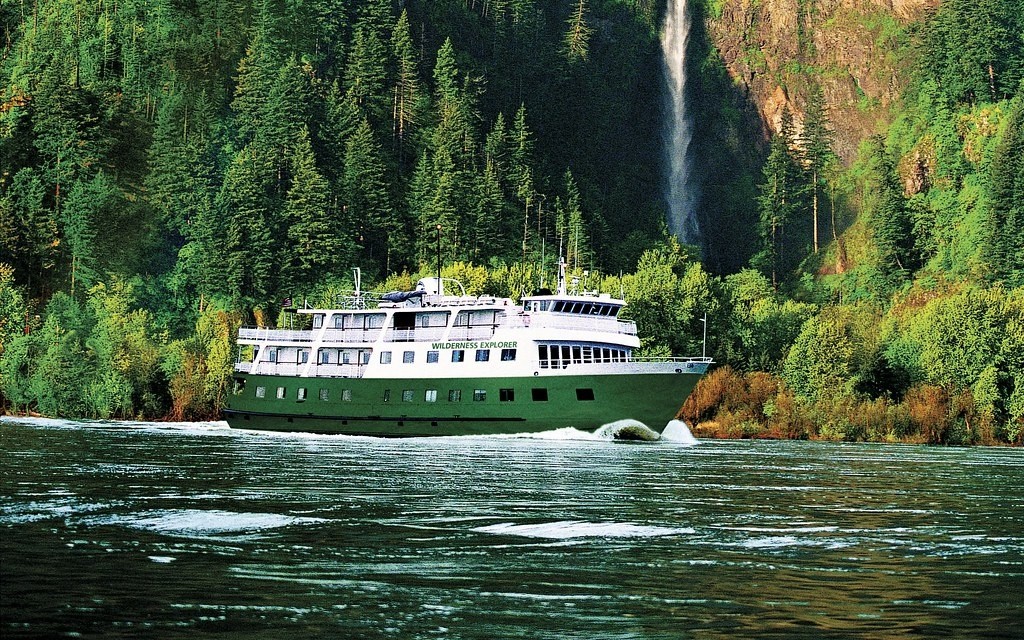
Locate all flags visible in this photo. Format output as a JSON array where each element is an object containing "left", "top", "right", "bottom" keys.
[{"left": 281, "top": 293, "right": 292, "bottom": 307}]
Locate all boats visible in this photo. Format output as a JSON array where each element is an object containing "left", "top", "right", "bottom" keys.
[{"left": 222, "top": 221, "right": 718, "bottom": 438}]
